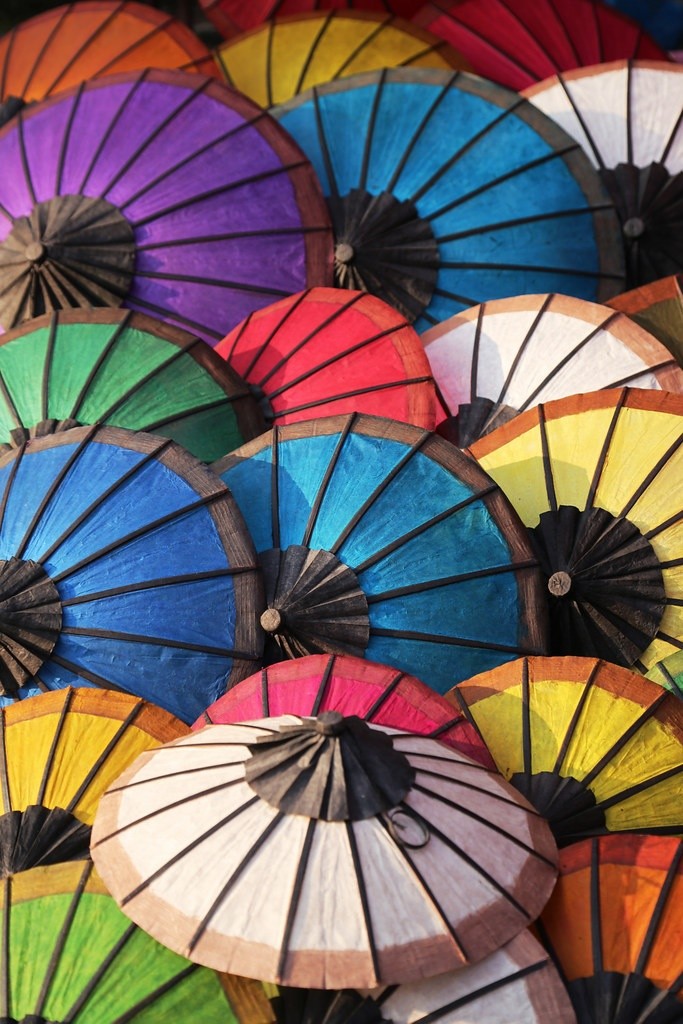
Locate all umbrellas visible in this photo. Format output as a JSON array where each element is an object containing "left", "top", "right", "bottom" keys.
[{"left": 2, "top": 0, "right": 683, "bottom": 1024}]
